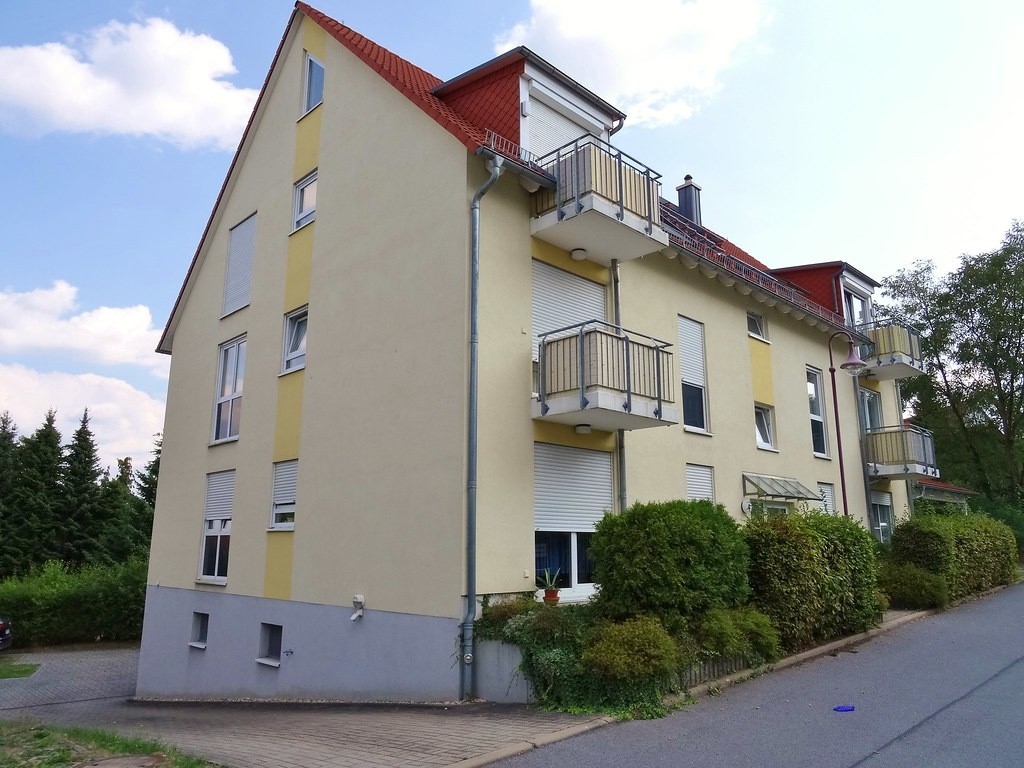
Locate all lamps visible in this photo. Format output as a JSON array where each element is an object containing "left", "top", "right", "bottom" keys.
[
  {"left": 575, "top": 425, "right": 592, "bottom": 435},
  {"left": 881, "top": 478, "right": 890, "bottom": 484},
  {"left": 865, "top": 373, "right": 876, "bottom": 381},
  {"left": 571, "top": 250, "right": 586, "bottom": 261}
]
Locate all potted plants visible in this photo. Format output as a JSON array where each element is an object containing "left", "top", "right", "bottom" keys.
[{"left": 537, "top": 568, "right": 562, "bottom": 605}]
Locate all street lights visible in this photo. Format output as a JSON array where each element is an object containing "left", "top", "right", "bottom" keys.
[{"left": 829, "top": 330, "right": 869, "bottom": 522}]
